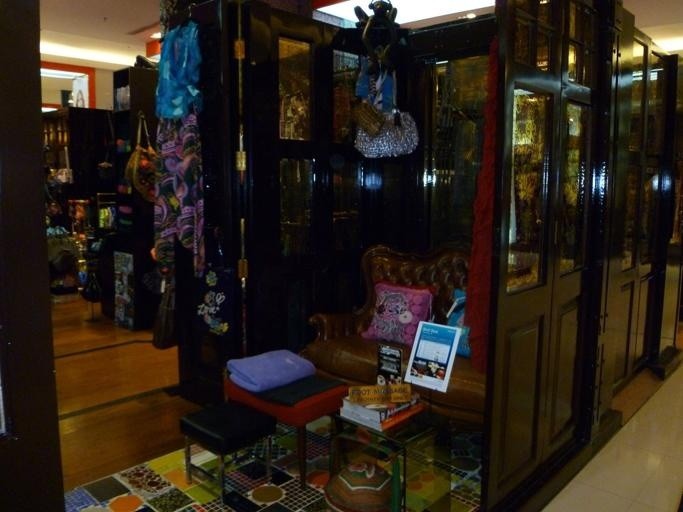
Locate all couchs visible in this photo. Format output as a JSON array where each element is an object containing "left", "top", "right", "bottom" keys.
[{"left": 298, "top": 242, "right": 575, "bottom": 425}]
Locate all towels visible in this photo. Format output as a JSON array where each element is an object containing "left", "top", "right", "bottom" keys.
[{"left": 226, "top": 349, "right": 317, "bottom": 394}]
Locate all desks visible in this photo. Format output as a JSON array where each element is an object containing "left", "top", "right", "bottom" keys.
[{"left": 328, "top": 406, "right": 451, "bottom": 512}]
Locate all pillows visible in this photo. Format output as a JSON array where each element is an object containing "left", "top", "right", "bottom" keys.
[
  {"left": 361, "top": 283, "right": 433, "bottom": 348},
  {"left": 447, "top": 289, "right": 472, "bottom": 358}
]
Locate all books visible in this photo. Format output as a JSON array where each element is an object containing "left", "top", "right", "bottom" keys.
[{"left": 340, "top": 391, "right": 424, "bottom": 431}]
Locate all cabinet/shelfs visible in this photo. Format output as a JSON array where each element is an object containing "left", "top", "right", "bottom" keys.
[
  {"left": 42, "top": 106, "right": 115, "bottom": 237},
  {"left": 97, "top": 67, "right": 161, "bottom": 332},
  {"left": 406, "top": 11, "right": 495, "bottom": 256}
]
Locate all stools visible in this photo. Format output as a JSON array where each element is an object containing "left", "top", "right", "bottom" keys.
[
  {"left": 179, "top": 400, "right": 277, "bottom": 506},
  {"left": 224, "top": 362, "right": 352, "bottom": 492}
]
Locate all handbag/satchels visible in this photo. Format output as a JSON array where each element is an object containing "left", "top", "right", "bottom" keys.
[
  {"left": 124, "top": 113, "right": 159, "bottom": 203},
  {"left": 355, "top": 98, "right": 384, "bottom": 139},
  {"left": 354, "top": 100, "right": 419, "bottom": 160}
]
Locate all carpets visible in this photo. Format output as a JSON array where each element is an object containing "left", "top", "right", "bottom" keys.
[{"left": 65, "top": 417, "right": 483, "bottom": 512}]
